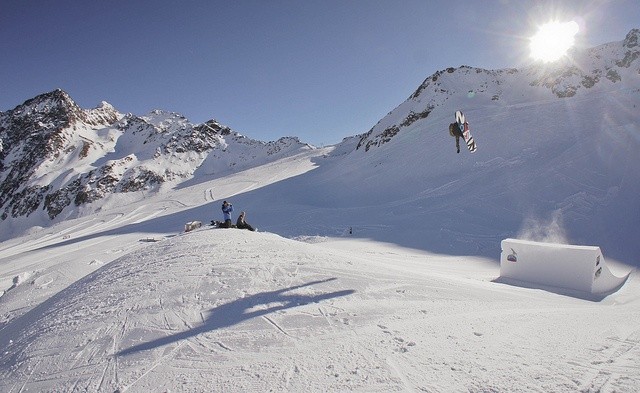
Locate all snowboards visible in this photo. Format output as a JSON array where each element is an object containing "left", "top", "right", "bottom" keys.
[{"left": 455, "top": 110, "right": 477, "bottom": 153}]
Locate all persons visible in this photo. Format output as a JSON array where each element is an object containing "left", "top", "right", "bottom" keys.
[
  {"left": 216, "top": 201, "right": 234, "bottom": 228},
  {"left": 236, "top": 211, "right": 257, "bottom": 231},
  {"left": 449, "top": 121, "right": 468, "bottom": 153}
]
